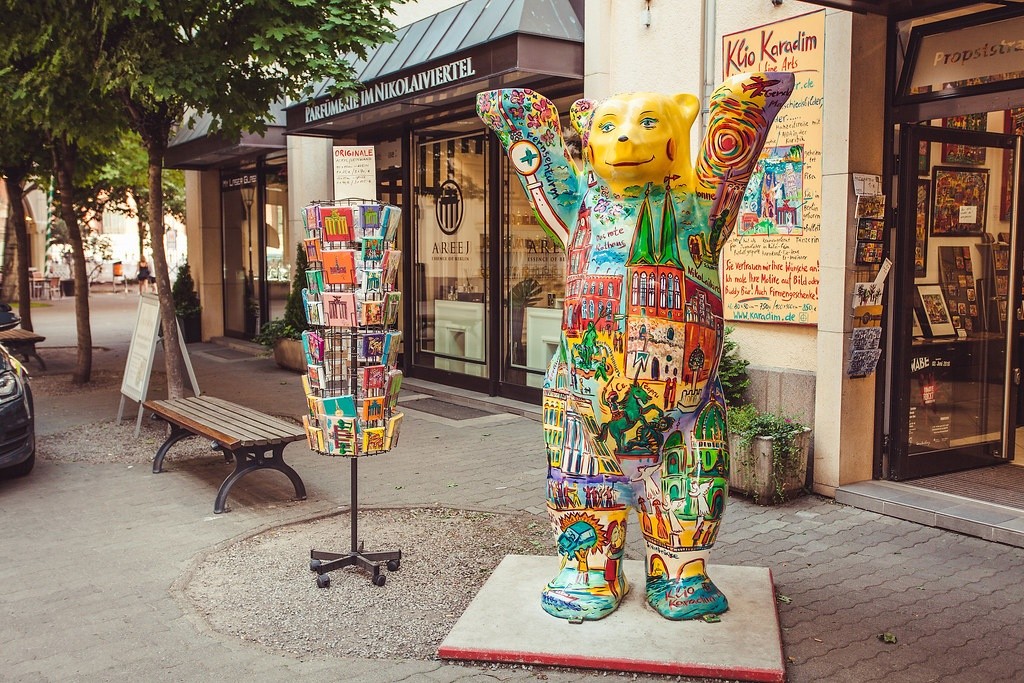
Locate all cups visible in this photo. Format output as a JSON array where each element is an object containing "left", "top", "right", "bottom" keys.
[
  {"left": 548, "top": 293, "right": 556, "bottom": 308},
  {"left": 553, "top": 298, "right": 564, "bottom": 308}
]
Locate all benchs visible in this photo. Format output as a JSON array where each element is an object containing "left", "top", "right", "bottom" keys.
[
  {"left": 0, "top": 328, "right": 46, "bottom": 364},
  {"left": 142, "top": 396, "right": 307, "bottom": 514}
]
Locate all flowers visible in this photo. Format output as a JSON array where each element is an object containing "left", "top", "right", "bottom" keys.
[{"left": 726, "top": 403, "right": 812, "bottom": 505}]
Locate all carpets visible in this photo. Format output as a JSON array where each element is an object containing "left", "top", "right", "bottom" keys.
[{"left": 903, "top": 443, "right": 1024, "bottom": 510}]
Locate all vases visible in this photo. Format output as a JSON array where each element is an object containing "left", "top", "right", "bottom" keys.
[{"left": 730, "top": 427, "right": 812, "bottom": 504}]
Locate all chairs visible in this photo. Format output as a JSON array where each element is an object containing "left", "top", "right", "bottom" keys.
[{"left": 51, "top": 276, "right": 60, "bottom": 297}]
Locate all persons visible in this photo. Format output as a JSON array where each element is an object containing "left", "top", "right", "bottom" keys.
[{"left": 136, "top": 255, "right": 151, "bottom": 295}]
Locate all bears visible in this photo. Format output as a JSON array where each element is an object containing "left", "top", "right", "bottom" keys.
[{"left": 477, "top": 70, "right": 793, "bottom": 623}]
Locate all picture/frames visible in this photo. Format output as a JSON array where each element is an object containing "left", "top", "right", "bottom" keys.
[
  {"left": 918, "top": 85, "right": 933, "bottom": 175},
  {"left": 930, "top": 166, "right": 990, "bottom": 238},
  {"left": 914, "top": 179, "right": 931, "bottom": 278},
  {"left": 915, "top": 284, "right": 957, "bottom": 339}
]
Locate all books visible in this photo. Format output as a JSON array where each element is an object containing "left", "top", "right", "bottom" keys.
[
  {"left": 855, "top": 195, "right": 886, "bottom": 218},
  {"left": 851, "top": 327, "right": 882, "bottom": 355},
  {"left": 851, "top": 240, "right": 882, "bottom": 264},
  {"left": 851, "top": 283, "right": 884, "bottom": 309},
  {"left": 853, "top": 172, "right": 883, "bottom": 197},
  {"left": 851, "top": 305, "right": 883, "bottom": 336},
  {"left": 847, "top": 349, "right": 882, "bottom": 374},
  {"left": 855, "top": 218, "right": 884, "bottom": 242}
]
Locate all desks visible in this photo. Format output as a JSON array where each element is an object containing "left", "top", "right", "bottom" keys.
[{"left": 30, "top": 278, "right": 51, "bottom": 298}]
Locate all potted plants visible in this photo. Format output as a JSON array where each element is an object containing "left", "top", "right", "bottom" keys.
[
  {"left": 252, "top": 243, "right": 309, "bottom": 374},
  {"left": 159, "top": 264, "right": 200, "bottom": 342}
]
